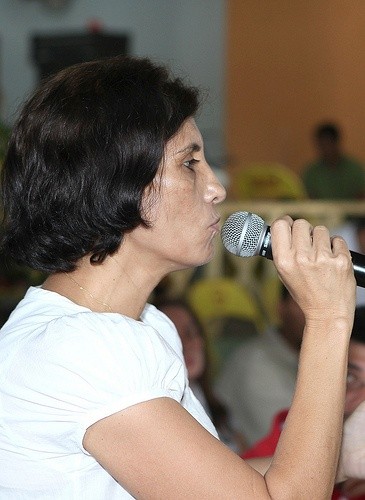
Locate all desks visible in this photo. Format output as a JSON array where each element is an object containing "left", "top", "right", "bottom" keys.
[{"left": 169, "top": 200, "right": 365, "bottom": 299}]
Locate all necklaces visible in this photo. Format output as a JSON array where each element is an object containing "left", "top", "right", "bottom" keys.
[{"left": 61, "top": 269, "right": 115, "bottom": 315}]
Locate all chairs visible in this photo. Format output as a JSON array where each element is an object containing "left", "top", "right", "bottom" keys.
[
  {"left": 232, "top": 161, "right": 307, "bottom": 199},
  {"left": 188, "top": 277, "right": 266, "bottom": 386}
]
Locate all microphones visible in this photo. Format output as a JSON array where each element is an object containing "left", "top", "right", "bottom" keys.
[{"left": 221, "top": 212, "right": 365, "bottom": 289}]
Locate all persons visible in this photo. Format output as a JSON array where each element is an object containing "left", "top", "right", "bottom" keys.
[
  {"left": 239, "top": 303, "right": 364, "bottom": 500},
  {"left": 300, "top": 122, "right": 362, "bottom": 200},
  {"left": 324, "top": 214, "right": 365, "bottom": 306},
  {"left": 213, "top": 277, "right": 310, "bottom": 450},
  {"left": 0, "top": 54, "right": 358, "bottom": 500},
  {"left": 151, "top": 295, "right": 217, "bottom": 426}
]
